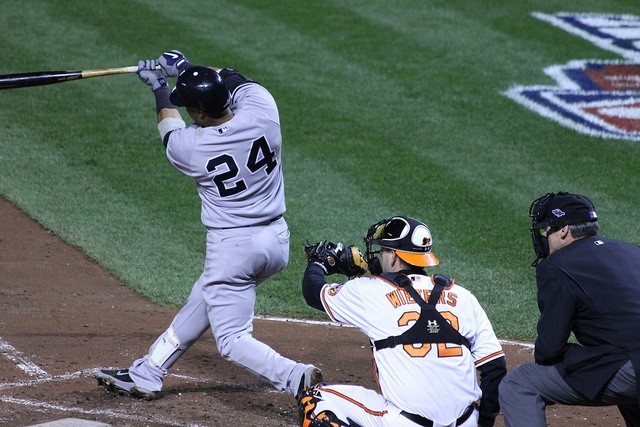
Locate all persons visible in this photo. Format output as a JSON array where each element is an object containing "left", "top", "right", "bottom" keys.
[
  {"left": 497, "top": 191, "right": 635, "bottom": 425},
  {"left": 299, "top": 215, "right": 507, "bottom": 427},
  {"left": 94, "top": 50, "right": 323, "bottom": 400}
]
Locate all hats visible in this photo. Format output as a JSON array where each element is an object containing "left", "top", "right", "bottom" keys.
[{"left": 528, "top": 191, "right": 598, "bottom": 267}]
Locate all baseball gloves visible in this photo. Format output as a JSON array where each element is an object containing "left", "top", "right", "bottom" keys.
[{"left": 301, "top": 237, "right": 368, "bottom": 281}]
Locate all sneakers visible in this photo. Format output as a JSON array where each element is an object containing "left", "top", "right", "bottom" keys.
[
  {"left": 94, "top": 369, "right": 163, "bottom": 400},
  {"left": 297, "top": 367, "right": 323, "bottom": 412}
]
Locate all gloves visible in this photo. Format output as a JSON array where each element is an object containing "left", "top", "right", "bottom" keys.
[
  {"left": 136, "top": 60, "right": 169, "bottom": 91},
  {"left": 158, "top": 49, "right": 191, "bottom": 77}
]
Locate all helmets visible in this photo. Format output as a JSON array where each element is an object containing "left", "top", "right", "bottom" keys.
[
  {"left": 363, "top": 216, "right": 440, "bottom": 275},
  {"left": 170, "top": 67, "right": 232, "bottom": 112}
]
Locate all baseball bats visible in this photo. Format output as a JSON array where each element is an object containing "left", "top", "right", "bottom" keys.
[{"left": 0, "top": 63, "right": 162, "bottom": 90}]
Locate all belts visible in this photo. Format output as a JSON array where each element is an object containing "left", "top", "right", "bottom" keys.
[{"left": 401, "top": 404, "right": 473, "bottom": 427}]
